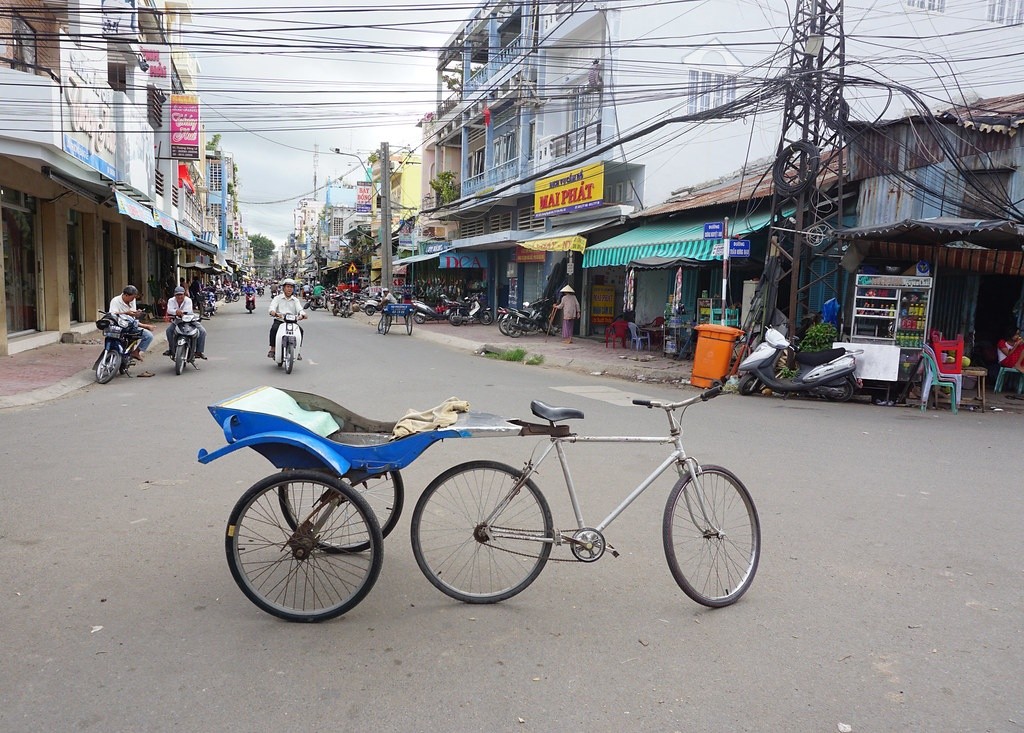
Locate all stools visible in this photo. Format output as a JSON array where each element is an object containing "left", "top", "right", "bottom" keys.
[{"left": 931, "top": 329, "right": 965, "bottom": 375}]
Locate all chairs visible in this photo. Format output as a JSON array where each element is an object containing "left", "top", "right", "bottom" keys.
[
  {"left": 606, "top": 320, "right": 628, "bottom": 349},
  {"left": 918, "top": 340, "right": 962, "bottom": 415},
  {"left": 627, "top": 321, "right": 652, "bottom": 352}
]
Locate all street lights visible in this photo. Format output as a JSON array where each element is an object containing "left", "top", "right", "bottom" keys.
[{"left": 329, "top": 142, "right": 395, "bottom": 297}]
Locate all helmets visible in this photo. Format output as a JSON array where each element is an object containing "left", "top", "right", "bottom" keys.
[{"left": 281, "top": 279, "right": 295, "bottom": 286}]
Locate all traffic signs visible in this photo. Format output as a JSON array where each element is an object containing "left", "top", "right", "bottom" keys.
[
  {"left": 703, "top": 222, "right": 724, "bottom": 239},
  {"left": 711, "top": 243, "right": 725, "bottom": 256},
  {"left": 729, "top": 239, "right": 752, "bottom": 258}
]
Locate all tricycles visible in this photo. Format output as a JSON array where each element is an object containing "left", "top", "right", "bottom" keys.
[{"left": 377, "top": 303, "right": 414, "bottom": 336}]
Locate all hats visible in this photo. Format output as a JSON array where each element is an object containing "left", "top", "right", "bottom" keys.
[
  {"left": 174, "top": 286, "right": 185, "bottom": 296},
  {"left": 559, "top": 285, "right": 574, "bottom": 293}
]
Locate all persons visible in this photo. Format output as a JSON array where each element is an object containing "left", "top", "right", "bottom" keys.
[
  {"left": 162, "top": 285, "right": 207, "bottom": 360},
  {"left": 266, "top": 277, "right": 308, "bottom": 360},
  {"left": 182, "top": 271, "right": 370, "bottom": 317},
  {"left": 997, "top": 324, "right": 1024, "bottom": 374},
  {"left": 379, "top": 287, "right": 398, "bottom": 308},
  {"left": 109, "top": 283, "right": 156, "bottom": 362},
  {"left": 555, "top": 283, "right": 581, "bottom": 342}
]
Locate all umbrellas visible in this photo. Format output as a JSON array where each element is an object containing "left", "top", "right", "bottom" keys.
[
  {"left": 830, "top": 213, "right": 1023, "bottom": 253},
  {"left": 178, "top": 261, "right": 224, "bottom": 274}
]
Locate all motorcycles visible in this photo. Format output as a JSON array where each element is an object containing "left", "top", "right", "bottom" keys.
[
  {"left": 203, "top": 281, "right": 495, "bottom": 326},
  {"left": 165, "top": 310, "right": 211, "bottom": 375},
  {"left": 737, "top": 324, "right": 864, "bottom": 402},
  {"left": 267, "top": 301, "right": 310, "bottom": 374},
  {"left": 497, "top": 297, "right": 558, "bottom": 337},
  {"left": 90, "top": 309, "right": 143, "bottom": 384}
]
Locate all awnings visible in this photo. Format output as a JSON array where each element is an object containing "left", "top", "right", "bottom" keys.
[
  {"left": 190, "top": 236, "right": 219, "bottom": 255},
  {"left": 391, "top": 247, "right": 455, "bottom": 266},
  {"left": 517, "top": 217, "right": 623, "bottom": 241},
  {"left": 625, "top": 255, "right": 720, "bottom": 272},
  {"left": 580, "top": 198, "right": 811, "bottom": 270}
]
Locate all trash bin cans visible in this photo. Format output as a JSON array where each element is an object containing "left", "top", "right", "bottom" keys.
[{"left": 688, "top": 322, "right": 745, "bottom": 390}]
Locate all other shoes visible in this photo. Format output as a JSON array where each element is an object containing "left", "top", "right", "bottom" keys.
[
  {"left": 196, "top": 351, "right": 207, "bottom": 359},
  {"left": 268, "top": 350, "right": 275, "bottom": 358},
  {"left": 137, "top": 370, "right": 155, "bottom": 377},
  {"left": 297, "top": 354, "right": 302, "bottom": 360},
  {"left": 163, "top": 350, "right": 172, "bottom": 356}
]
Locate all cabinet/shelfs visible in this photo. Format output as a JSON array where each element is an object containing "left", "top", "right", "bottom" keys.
[{"left": 850, "top": 272, "right": 932, "bottom": 352}]
